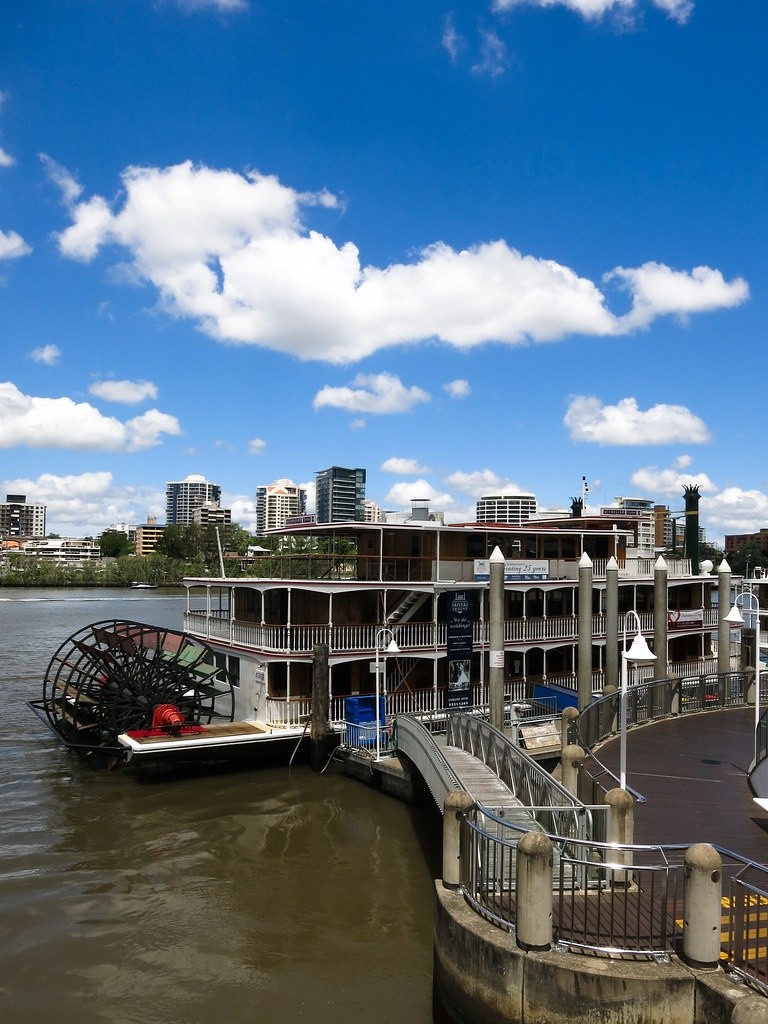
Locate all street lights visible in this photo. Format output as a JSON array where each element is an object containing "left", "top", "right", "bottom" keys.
[
  {"left": 376, "top": 629, "right": 401, "bottom": 761},
  {"left": 722, "top": 592, "right": 761, "bottom": 765},
  {"left": 620, "top": 610, "right": 659, "bottom": 790}
]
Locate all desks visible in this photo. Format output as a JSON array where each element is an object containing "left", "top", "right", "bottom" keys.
[{"left": 368, "top": 563, "right": 394, "bottom": 581}]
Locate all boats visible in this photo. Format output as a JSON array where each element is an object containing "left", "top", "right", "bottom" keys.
[{"left": 130, "top": 581, "right": 160, "bottom": 589}]
